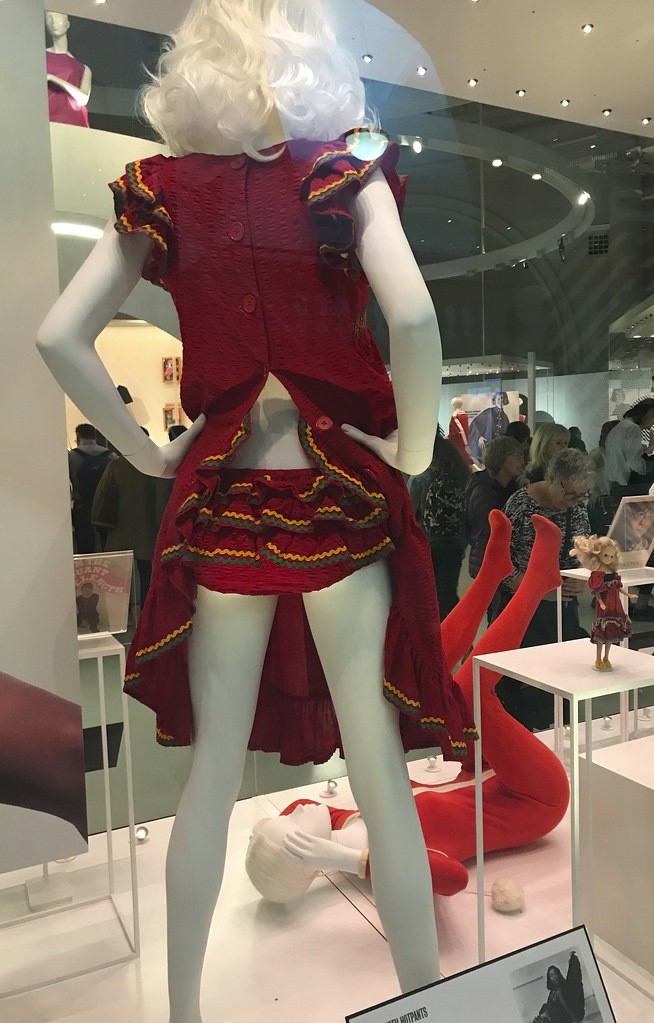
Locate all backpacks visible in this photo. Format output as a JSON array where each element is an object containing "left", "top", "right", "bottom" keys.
[
  {"left": 420, "top": 463, "right": 470, "bottom": 539},
  {"left": 71, "top": 448, "right": 114, "bottom": 517}
]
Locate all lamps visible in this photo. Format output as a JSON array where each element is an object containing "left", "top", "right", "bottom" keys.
[{"left": 558, "top": 238, "right": 568, "bottom": 265}]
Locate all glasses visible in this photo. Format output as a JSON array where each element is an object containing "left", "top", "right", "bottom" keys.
[
  {"left": 508, "top": 452, "right": 525, "bottom": 458},
  {"left": 554, "top": 471, "right": 592, "bottom": 502}
]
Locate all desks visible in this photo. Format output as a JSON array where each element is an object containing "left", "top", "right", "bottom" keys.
[
  {"left": 555, "top": 564, "right": 654, "bottom": 751},
  {"left": 473, "top": 637, "right": 654, "bottom": 969},
  {"left": 0, "top": 632, "right": 139, "bottom": 1000}
]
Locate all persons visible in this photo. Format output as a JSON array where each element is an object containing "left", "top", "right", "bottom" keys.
[
  {"left": 611, "top": 501, "right": 654, "bottom": 551},
  {"left": 45, "top": 12, "right": 92, "bottom": 128},
  {"left": 407, "top": 397, "right": 654, "bottom": 732},
  {"left": 611, "top": 389, "right": 632, "bottom": 420},
  {"left": 245, "top": 509, "right": 570, "bottom": 903},
  {"left": 470, "top": 392, "right": 509, "bottom": 460},
  {"left": 569, "top": 534, "right": 639, "bottom": 669},
  {"left": 66, "top": 421, "right": 190, "bottom": 626},
  {"left": 29, "top": 0, "right": 449, "bottom": 1023},
  {"left": 76, "top": 582, "right": 101, "bottom": 633},
  {"left": 448, "top": 397, "right": 482, "bottom": 473}
]
